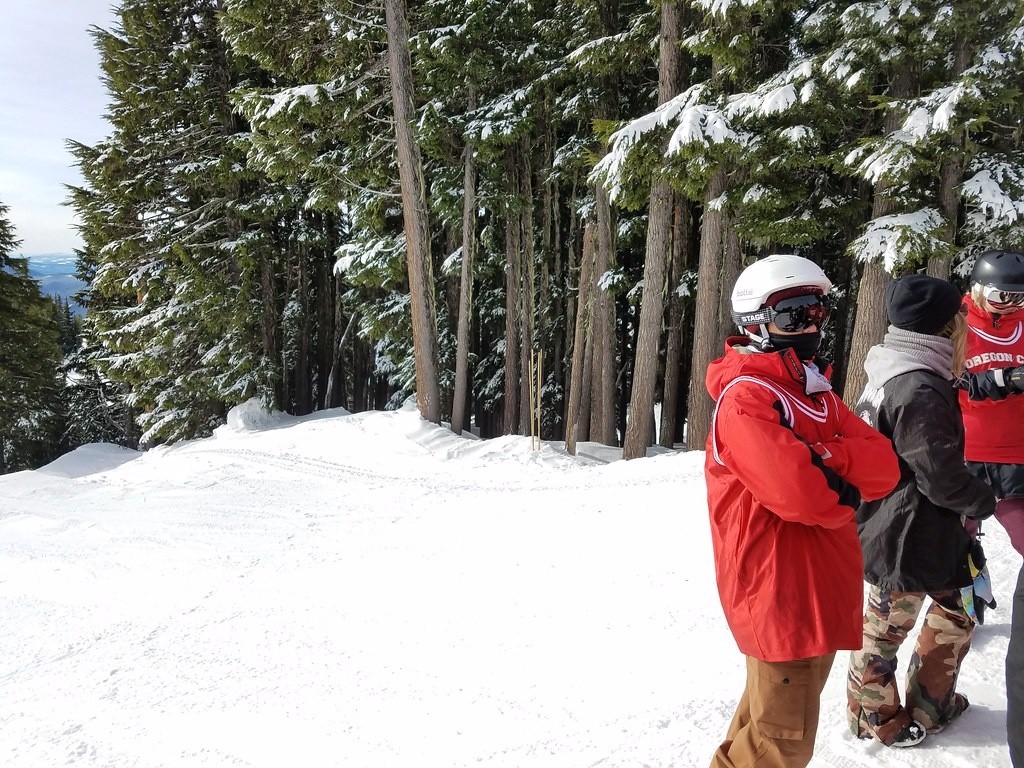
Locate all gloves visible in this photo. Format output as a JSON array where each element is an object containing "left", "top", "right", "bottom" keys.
[
  {"left": 1003, "top": 364, "right": 1024, "bottom": 395},
  {"left": 965, "top": 483, "right": 996, "bottom": 521}
]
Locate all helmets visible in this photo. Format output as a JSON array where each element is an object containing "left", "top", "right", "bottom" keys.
[
  {"left": 970, "top": 251, "right": 1024, "bottom": 293},
  {"left": 731, "top": 254, "right": 830, "bottom": 337}
]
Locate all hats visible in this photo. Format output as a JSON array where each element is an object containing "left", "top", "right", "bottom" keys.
[{"left": 886, "top": 276, "right": 962, "bottom": 335}]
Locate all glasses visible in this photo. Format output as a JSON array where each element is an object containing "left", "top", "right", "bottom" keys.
[
  {"left": 769, "top": 293, "right": 831, "bottom": 333},
  {"left": 985, "top": 288, "right": 1024, "bottom": 311}
]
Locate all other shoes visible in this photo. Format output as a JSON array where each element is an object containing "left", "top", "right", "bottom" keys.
[
  {"left": 927, "top": 693, "right": 968, "bottom": 734},
  {"left": 863, "top": 719, "right": 926, "bottom": 747}
]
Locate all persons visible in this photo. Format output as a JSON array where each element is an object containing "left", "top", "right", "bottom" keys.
[
  {"left": 704, "top": 254, "right": 901, "bottom": 768},
  {"left": 845, "top": 274, "right": 997, "bottom": 747},
  {"left": 953, "top": 250, "right": 1024, "bottom": 558}
]
